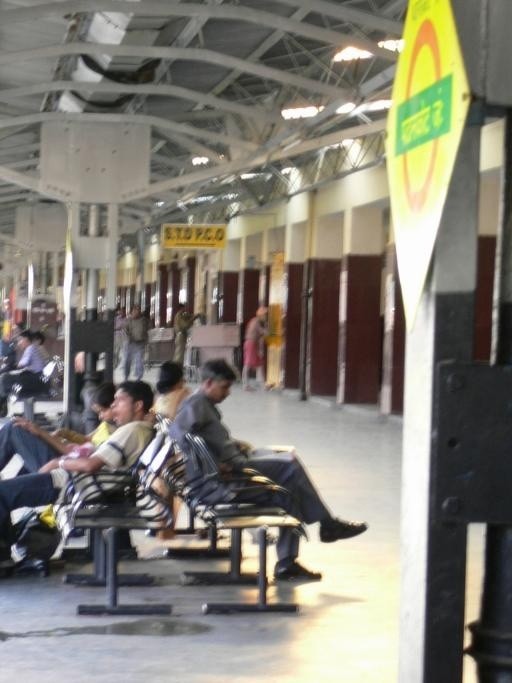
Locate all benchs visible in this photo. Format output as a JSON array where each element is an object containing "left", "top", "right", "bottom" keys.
[
  {"left": 57, "top": 414, "right": 308, "bottom": 616},
  {"left": 10, "top": 355, "right": 72, "bottom": 432}
]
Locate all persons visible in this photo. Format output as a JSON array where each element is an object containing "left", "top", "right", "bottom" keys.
[
  {"left": 172, "top": 302, "right": 200, "bottom": 365},
  {"left": 113, "top": 305, "right": 127, "bottom": 366},
  {"left": 0, "top": 327, "right": 190, "bottom": 580},
  {"left": 242, "top": 306, "right": 275, "bottom": 391},
  {"left": 169, "top": 359, "right": 367, "bottom": 581},
  {"left": 123, "top": 306, "right": 149, "bottom": 380}
]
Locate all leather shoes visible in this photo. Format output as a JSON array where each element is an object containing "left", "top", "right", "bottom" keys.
[
  {"left": 318, "top": 518, "right": 367, "bottom": 542},
  {"left": 274, "top": 557, "right": 321, "bottom": 582}
]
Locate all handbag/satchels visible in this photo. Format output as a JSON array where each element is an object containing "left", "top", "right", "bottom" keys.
[{"left": 14, "top": 507, "right": 61, "bottom": 561}]
